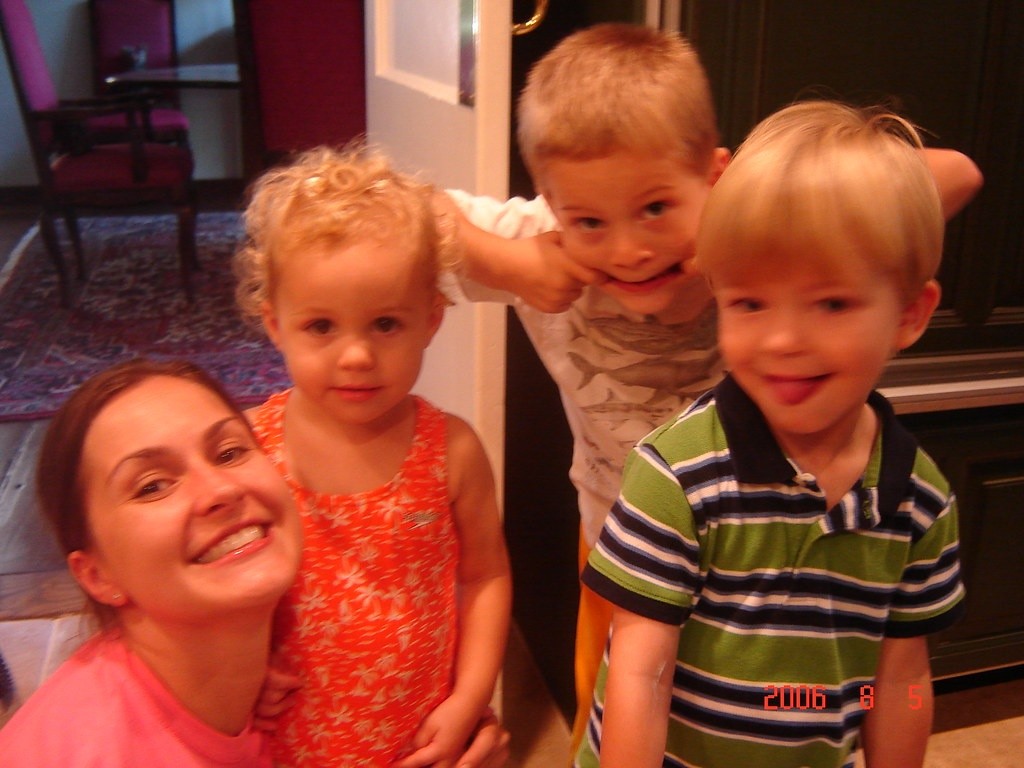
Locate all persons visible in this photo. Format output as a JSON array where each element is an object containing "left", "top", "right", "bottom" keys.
[
  {"left": 416, "top": 22, "right": 981, "bottom": 742},
  {"left": 2, "top": 143, "right": 511, "bottom": 768},
  {"left": 573, "top": 102, "right": 963, "bottom": 768}
]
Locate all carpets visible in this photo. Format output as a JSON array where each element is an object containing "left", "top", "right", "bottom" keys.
[{"left": 0, "top": 210, "right": 293, "bottom": 423}]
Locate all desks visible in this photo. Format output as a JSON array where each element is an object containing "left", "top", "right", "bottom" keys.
[{"left": 102, "top": 65, "right": 246, "bottom": 89}]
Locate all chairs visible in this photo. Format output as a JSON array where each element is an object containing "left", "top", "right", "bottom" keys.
[
  {"left": 0, "top": 0, "right": 198, "bottom": 307},
  {"left": 84, "top": 0, "right": 190, "bottom": 146}
]
